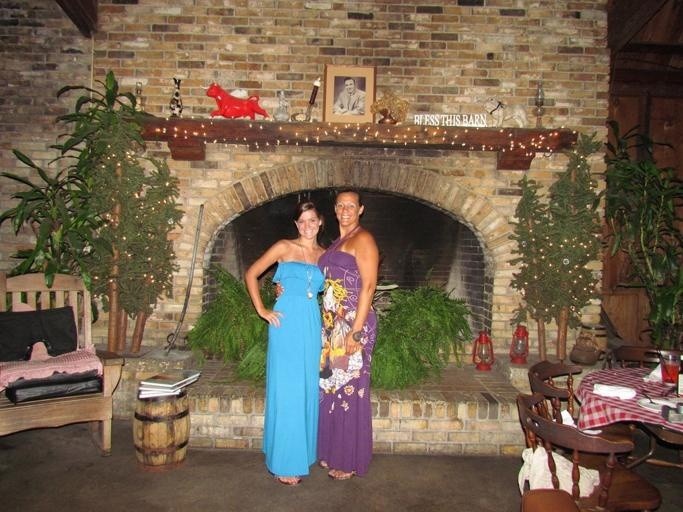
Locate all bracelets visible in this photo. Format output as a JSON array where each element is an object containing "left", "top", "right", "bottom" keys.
[{"left": 350, "top": 330, "right": 361, "bottom": 342}]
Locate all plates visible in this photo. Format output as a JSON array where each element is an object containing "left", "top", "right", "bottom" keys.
[{"left": 637, "top": 397, "right": 676, "bottom": 413}]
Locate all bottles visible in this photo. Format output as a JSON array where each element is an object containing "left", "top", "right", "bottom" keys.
[
  {"left": 169, "top": 76, "right": 182, "bottom": 118},
  {"left": 272, "top": 89, "right": 291, "bottom": 121},
  {"left": 678, "top": 354, "right": 683, "bottom": 398},
  {"left": 129, "top": 82, "right": 144, "bottom": 114}
]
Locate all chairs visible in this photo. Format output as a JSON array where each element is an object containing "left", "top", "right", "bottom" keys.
[
  {"left": 0, "top": 271, "right": 124, "bottom": 458},
  {"left": 514, "top": 345, "right": 683, "bottom": 512}
]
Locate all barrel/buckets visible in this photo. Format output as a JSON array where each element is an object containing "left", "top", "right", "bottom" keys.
[
  {"left": 130, "top": 385, "right": 192, "bottom": 465},
  {"left": 582, "top": 324, "right": 609, "bottom": 355}
]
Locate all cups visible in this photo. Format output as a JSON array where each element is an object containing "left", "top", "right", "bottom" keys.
[{"left": 660, "top": 355, "right": 677, "bottom": 388}]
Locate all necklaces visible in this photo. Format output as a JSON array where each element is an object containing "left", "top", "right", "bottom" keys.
[{"left": 299, "top": 239, "right": 318, "bottom": 299}]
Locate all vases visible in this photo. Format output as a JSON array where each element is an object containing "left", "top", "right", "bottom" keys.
[{"left": 170, "top": 77, "right": 183, "bottom": 118}]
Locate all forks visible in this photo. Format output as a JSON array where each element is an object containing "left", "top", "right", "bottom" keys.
[{"left": 642, "top": 392, "right": 659, "bottom": 406}]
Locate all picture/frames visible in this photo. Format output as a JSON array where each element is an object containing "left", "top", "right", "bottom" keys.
[{"left": 323, "top": 65, "right": 374, "bottom": 124}]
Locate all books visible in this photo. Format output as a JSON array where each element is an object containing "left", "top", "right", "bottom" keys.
[{"left": 138, "top": 368, "right": 201, "bottom": 398}]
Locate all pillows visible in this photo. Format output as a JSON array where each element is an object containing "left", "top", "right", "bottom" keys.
[{"left": 0, "top": 345, "right": 103, "bottom": 394}]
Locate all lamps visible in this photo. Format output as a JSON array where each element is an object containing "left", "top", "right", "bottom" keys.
[
  {"left": 509, "top": 326, "right": 528, "bottom": 364},
  {"left": 473, "top": 331, "right": 494, "bottom": 371}
]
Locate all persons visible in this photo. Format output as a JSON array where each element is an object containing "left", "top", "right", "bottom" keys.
[
  {"left": 332, "top": 77, "right": 366, "bottom": 115},
  {"left": 317, "top": 190, "right": 380, "bottom": 481},
  {"left": 244, "top": 201, "right": 327, "bottom": 485}
]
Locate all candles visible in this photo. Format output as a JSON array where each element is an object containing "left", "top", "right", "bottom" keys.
[{"left": 314, "top": 77, "right": 321, "bottom": 87}]
